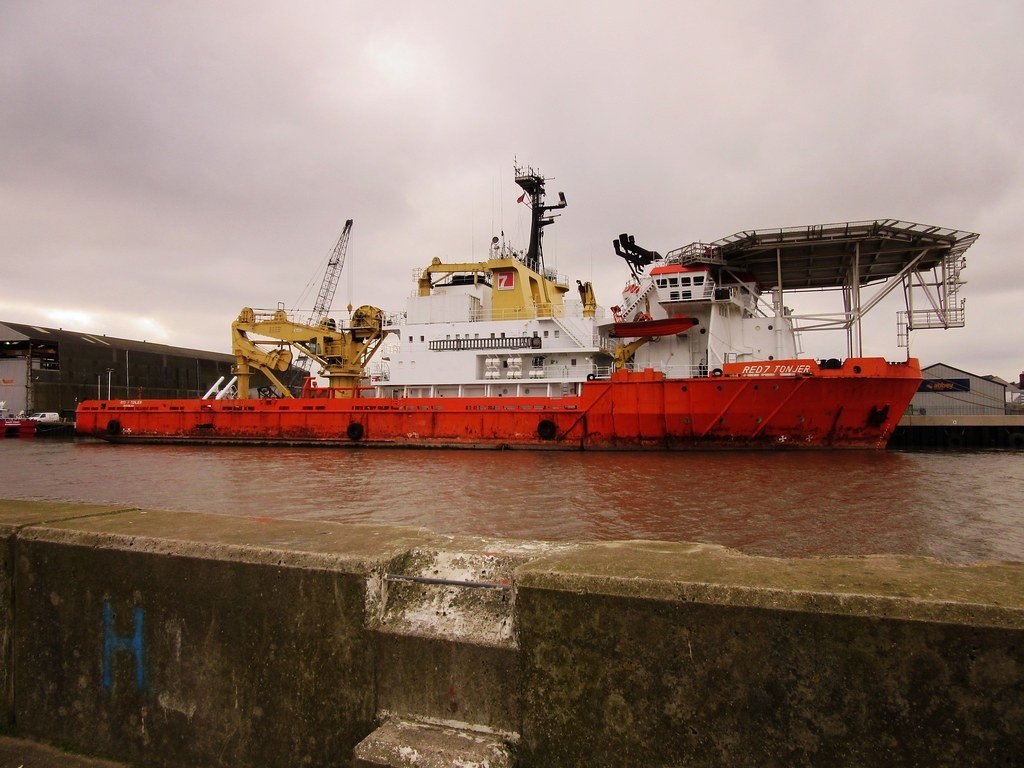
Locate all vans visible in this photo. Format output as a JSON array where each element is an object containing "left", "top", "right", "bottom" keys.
[{"left": 28, "top": 412, "right": 59, "bottom": 422}]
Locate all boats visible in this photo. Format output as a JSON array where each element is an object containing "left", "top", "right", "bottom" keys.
[
  {"left": 76, "top": 356, "right": 921, "bottom": 452},
  {"left": 331, "top": 162, "right": 795, "bottom": 401}
]
